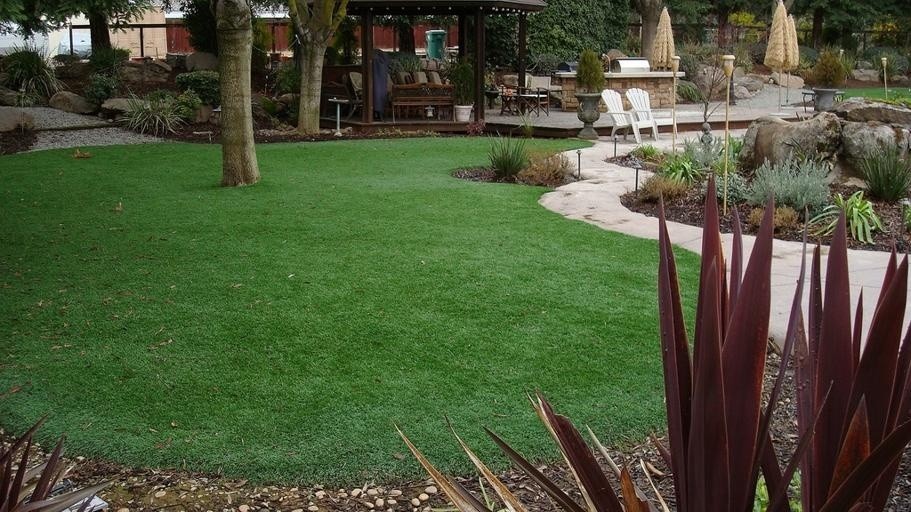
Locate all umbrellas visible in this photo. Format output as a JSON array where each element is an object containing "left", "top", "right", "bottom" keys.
[
  {"left": 653, "top": 6, "right": 675, "bottom": 72},
  {"left": 763, "top": 0, "right": 799, "bottom": 113}
]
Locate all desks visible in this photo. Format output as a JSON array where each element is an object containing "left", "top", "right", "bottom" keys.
[{"left": 802, "top": 91, "right": 846, "bottom": 113}]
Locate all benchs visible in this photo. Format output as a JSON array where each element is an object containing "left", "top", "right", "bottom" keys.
[
  {"left": 390, "top": 82, "right": 455, "bottom": 123},
  {"left": 322, "top": 81, "right": 362, "bottom": 117}
]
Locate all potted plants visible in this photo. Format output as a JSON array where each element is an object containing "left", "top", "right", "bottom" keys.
[
  {"left": 444, "top": 58, "right": 478, "bottom": 121},
  {"left": 575, "top": 49, "right": 608, "bottom": 140},
  {"left": 808, "top": 44, "right": 851, "bottom": 113}
]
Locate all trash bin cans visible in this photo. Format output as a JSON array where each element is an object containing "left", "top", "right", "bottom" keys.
[{"left": 424, "top": 30, "right": 447, "bottom": 60}]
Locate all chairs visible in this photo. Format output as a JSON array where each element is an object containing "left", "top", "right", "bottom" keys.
[
  {"left": 601, "top": 88, "right": 679, "bottom": 144},
  {"left": 345, "top": 71, "right": 443, "bottom": 121},
  {"left": 500, "top": 76, "right": 551, "bottom": 116}
]
